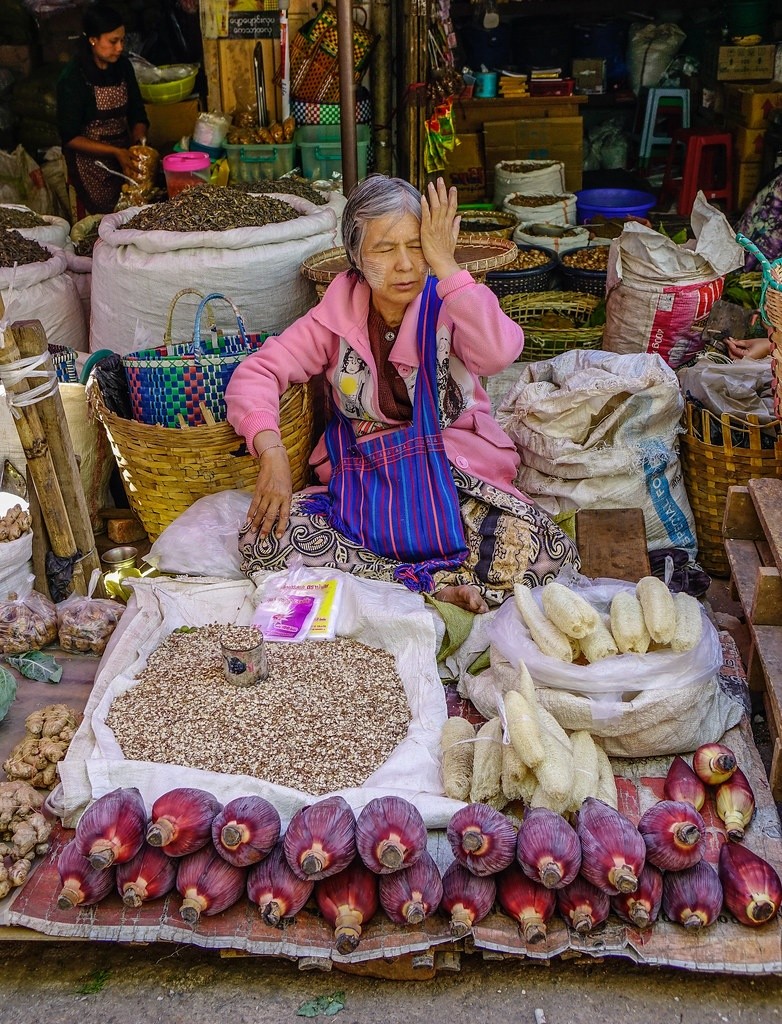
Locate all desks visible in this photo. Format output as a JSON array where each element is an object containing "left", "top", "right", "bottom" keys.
[{"left": 449, "top": 95, "right": 589, "bottom": 120}]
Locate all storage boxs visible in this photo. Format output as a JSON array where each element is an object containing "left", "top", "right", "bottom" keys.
[
  {"left": 225, "top": 131, "right": 298, "bottom": 181},
  {"left": 296, "top": 124, "right": 369, "bottom": 185},
  {"left": 705, "top": 41, "right": 776, "bottom": 84}
]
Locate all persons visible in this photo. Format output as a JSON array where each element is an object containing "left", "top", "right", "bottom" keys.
[
  {"left": 55, "top": 9, "right": 150, "bottom": 213},
  {"left": 220, "top": 167, "right": 586, "bottom": 616},
  {"left": 722, "top": 334, "right": 774, "bottom": 362}
]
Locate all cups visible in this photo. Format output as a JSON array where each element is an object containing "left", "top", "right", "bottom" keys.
[
  {"left": 220, "top": 625, "right": 269, "bottom": 687},
  {"left": 100, "top": 547, "right": 138, "bottom": 575}
]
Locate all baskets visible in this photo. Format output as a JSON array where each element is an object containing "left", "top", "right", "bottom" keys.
[
  {"left": 678, "top": 404, "right": 781, "bottom": 580},
  {"left": 140, "top": 63, "right": 198, "bottom": 104},
  {"left": 87, "top": 354, "right": 314, "bottom": 543},
  {"left": 764, "top": 258, "right": 781, "bottom": 331},
  {"left": 559, "top": 244, "right": 612, "bottom": 296},
  {"left": 496, "top": 291, "right": 605, "bottom": 362},
  {"left": 457, "top": 210, "right": 518, "bottom": 239},
  {"left": 486, "top": 244, "right": 559, "bottom": 296},
  {"left": 300, "top": 237, "right": 519, "bottom": 301},
  {"left": 291, "top": 96, "right": 374, "bottom": 125}
]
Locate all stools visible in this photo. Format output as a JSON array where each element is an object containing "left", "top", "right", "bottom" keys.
[
  {"left": 638, "top": 86, "right": 690, "bottom": 158},
  {"left": 659, "top": 127, "right": 734, "bottom": 218}
]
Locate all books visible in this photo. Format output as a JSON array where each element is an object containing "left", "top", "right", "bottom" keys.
[
  {"left": 492, "top": 67, "right": 528, "bottom": 78},
  {"left": 531, "top": 63, "right": 562, "bottom": 73}
]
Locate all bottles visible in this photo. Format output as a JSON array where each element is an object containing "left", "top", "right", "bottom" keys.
[{"left": 163, "top": 151, "right": 212, "bottom": 200}]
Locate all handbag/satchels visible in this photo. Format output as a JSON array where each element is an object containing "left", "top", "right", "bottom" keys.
[
  {"left": 0, "top": 573, "right": 56, "bottom": 655},
  {"left": 311, "top": 2, "right": 382, "bottom": 71},
  {"left": 48, "top": 341, "right": 80, "bottom": 383},
  {"left": 271, "top": 6, "right": 381, "bottom": 105},
  {"left": 123, "top": 287, "right": 277, "bottom": 427},
  {"left": 55, "top": 567, "right": 126, "bottom": 656},
  {"left": 304, "top": 427, "right": 468, "bottom": 589}
]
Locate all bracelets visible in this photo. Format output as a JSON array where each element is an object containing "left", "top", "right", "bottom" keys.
[{"left": 259, "top": 444, "right": 287, "bottom": 459}]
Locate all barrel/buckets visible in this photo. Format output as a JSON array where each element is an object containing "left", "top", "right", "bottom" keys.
[{"left": 575, "top": 188, "right": 656, "bottom": 225}]
